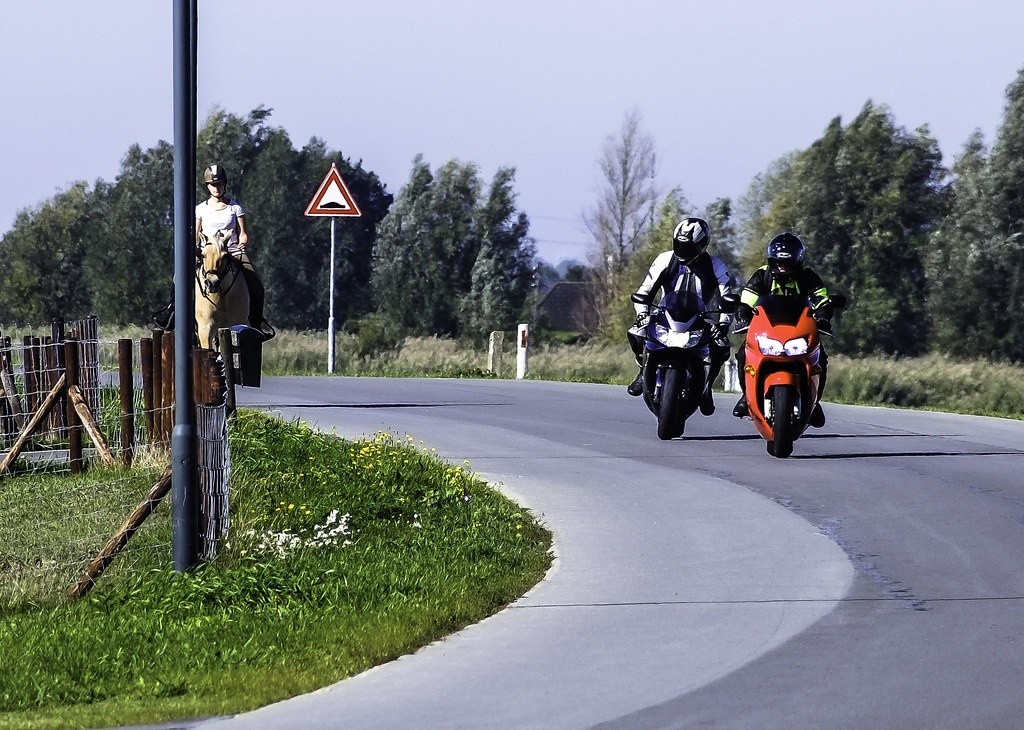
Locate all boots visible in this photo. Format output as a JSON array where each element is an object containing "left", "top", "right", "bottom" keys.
[
  {"left": 248, "top": 287, "right": 274, "bottom": 337},
  {"left": 153, "top": 283, "right": 175, "bottom": 327}
]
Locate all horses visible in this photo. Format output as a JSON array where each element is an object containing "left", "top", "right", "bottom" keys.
[{"left": 195, "top": 229, "right": 249, "bottom": 349}]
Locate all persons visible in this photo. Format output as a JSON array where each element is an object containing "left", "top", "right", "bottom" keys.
[
  {"left": 733, "top": 232, "right": 834, "bottom": 428},
  {"left": 625, "top": 218, "right": 742, "bottom": 416},
  {"left": 162, "top": 165, "right": 266, "bottom": 341}
]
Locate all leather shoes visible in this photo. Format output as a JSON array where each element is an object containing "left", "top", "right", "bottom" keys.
[
  {"left": 733, "top": 394, "right": 748, "bottom": 417},
  {"left": 809, "top": 403, "right": 825, "bottom": 428},
  {"left": 627, "top": 374, "right": 644, "bottom": 396},
  {"left": 700, "top": 389, "right": 714, "bottom": 415}
]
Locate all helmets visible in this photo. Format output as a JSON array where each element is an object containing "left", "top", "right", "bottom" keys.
[
  {"left": 673, "top": 218, "right": 711, "bottom": 265},
  {"left": 204, "top": 164, "right": 227, "bottom": 183},
  {"left": 767, "top": 233, "right": 806, "bottom": 284}
]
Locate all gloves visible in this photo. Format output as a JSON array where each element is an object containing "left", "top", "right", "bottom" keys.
[
  {"left": 635, "top": 312, "right": 649, "bottom": 327},
  {"left": 816, "top": 320, "right": 832, "bottom": 336},
  {"left": 708, "top": 322, "right": 729, "bottom": 339},
  {"left": 734, "top": 318, "right": 749, "bottom": 334}
]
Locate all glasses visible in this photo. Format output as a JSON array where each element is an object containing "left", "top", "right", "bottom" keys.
[{"left": 772, "top": 258, "right": 795, "bottom": 268}]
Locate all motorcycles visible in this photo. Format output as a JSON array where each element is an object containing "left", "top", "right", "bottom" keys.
[
  {"left": 631, "top": 293, "right": 736, "bottom": 440},
  {"left": 721, "top": 293, "right": 846, "bottom": 458}
]
